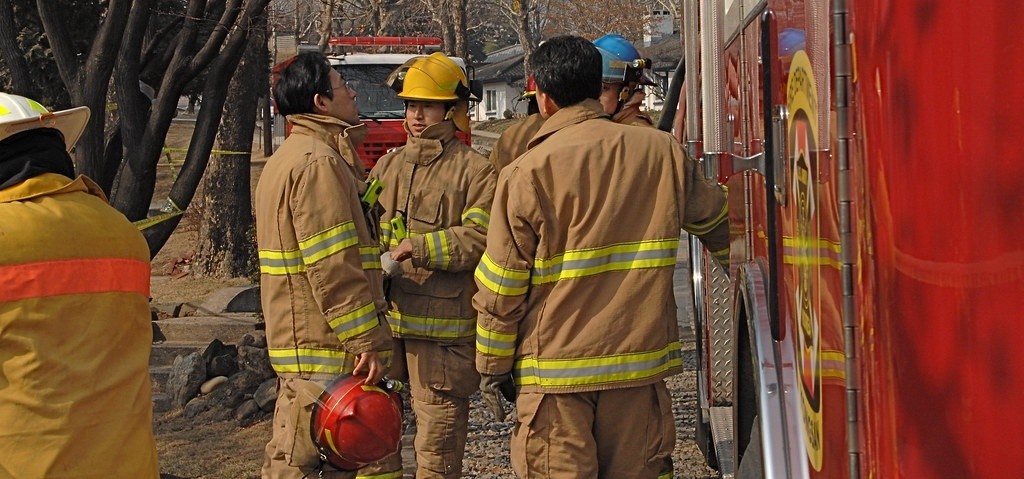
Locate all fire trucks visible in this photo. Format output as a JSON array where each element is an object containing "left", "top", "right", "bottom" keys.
[
  {"left": 656, "top": 1, "right": 1023, "bottom": 478},
  {"left": 273, "top": 34, "right": 485, "bottom": 185}
]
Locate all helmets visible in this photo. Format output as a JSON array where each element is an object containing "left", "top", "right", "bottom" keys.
[
  {"left": 309, "top": 370, "right": 403, "bottom": 472},
  {"left": 398, "top": 51, "right": 471, "bottom": 101},
  {"left": 1, "top": 88, "right": 92, "bottom": 155},
  {"left": 521, "top": 72, "right": 536, "bottom": 98},
  {"left": 591, "top": 33, "right": 658, "bottom": 86}
]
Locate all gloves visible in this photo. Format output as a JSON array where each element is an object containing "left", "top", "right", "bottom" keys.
[
  {"left": 380, "top": 251, "right": 401, "bottom": 303},
  {"left": 478, "top": 372, "right": 516, "bottom": 424}
]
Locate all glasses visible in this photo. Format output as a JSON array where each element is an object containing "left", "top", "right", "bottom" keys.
[{"left": 326, "top": 80, "right": 351, "bottom": 93}]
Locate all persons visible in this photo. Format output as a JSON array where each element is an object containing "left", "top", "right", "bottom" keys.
[
  {"left": 472, "top": 35, "right": 730, "bottom": 479},
  {"left": 255, "top": 52, "right": 394, "bottom": 479},
  {"left": 1, "top": 92, "right": 160, "bottom": 479},
  {"left": 355, "top": 33, "right": 658, "bottom": 479}
]
